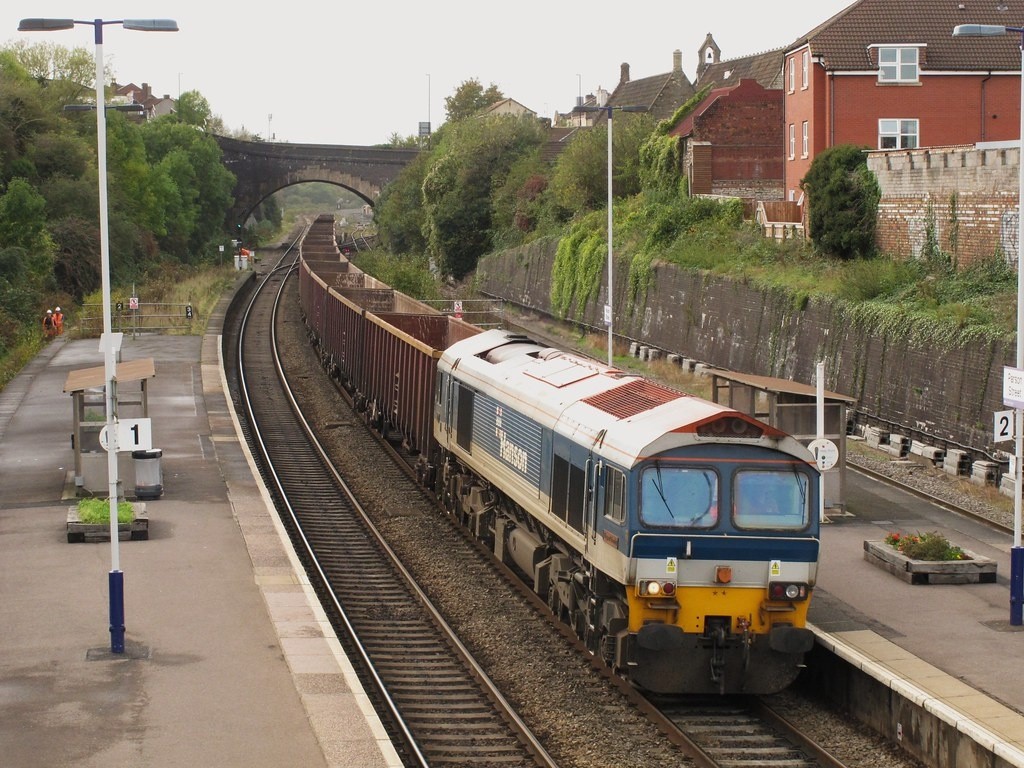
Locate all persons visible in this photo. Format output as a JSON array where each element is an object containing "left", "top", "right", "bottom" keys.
[
  {"left": 53, "top": 306, "right": 64, "bottom": 336},
  {"left": 745, "top": 480, "right": 779, "bottom": 516},
  {"left": 43, "top": 310, "right": 58, "bottom": 342}
]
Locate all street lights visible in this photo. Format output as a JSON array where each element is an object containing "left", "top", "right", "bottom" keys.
[
  {"left": 949, "top": 22, "right": 1023, "bottom": 627},
  {"left": 15, "top": 16, "right": 180, "bottom": 654},
  {"left": 574, "top": 104, "right": 648, "bottom": 368}
]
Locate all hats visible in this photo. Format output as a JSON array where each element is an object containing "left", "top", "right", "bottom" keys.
[
  {"left": 55, "top": 306, "right": 61, "bottom": 311},
  {"left": 46, "top": 309, "right": 52, "bottom": 314}
]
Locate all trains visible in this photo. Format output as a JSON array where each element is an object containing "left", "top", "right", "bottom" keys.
[{"left": 301, "top": 210, "right": 821, "bottom": 699}]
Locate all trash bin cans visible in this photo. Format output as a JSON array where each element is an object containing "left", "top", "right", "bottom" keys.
[{"left": 132, "top": 449, "right": 165, "bottom": 501}]
